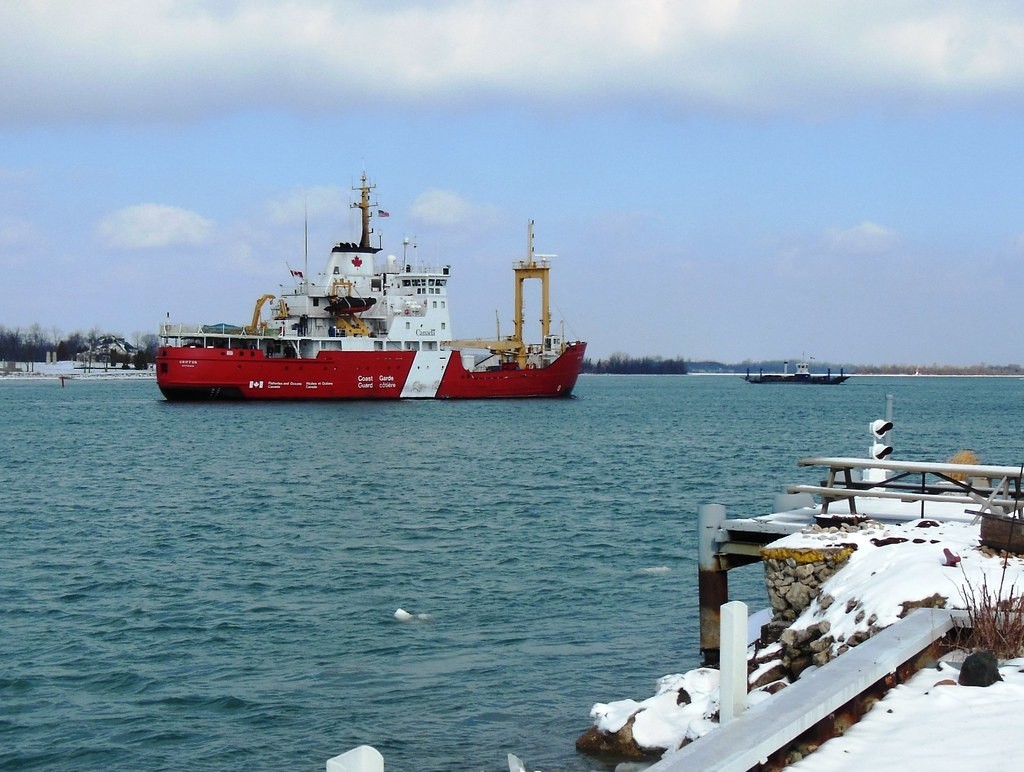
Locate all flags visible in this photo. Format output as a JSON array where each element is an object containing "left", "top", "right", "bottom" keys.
[{"left": 378, "top": 210, "right": 389, "bottom": 217}]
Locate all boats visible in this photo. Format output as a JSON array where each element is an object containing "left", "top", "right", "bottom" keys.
[
  {"left": 154, "top": 170, "right": 588, "bottom": 403},
  {"left": 739, "top": 352, "right": 850, "bottom": 385}
]
